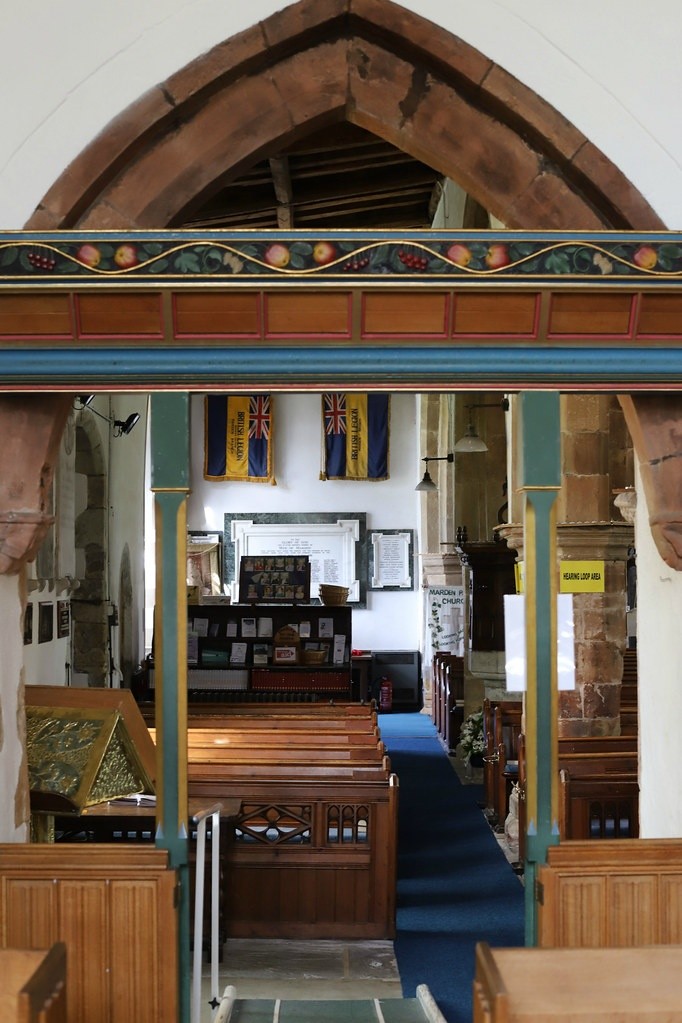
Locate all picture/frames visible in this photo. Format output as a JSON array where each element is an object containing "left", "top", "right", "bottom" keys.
[{"left": 239, "top": 555, "right": 311, "bottom": 604}]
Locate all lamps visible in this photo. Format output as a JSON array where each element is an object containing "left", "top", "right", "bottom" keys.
[
  {"left": 72, "top": 394, "right": 95, "bottom": 410},
  {"left": 415, "top": 454, "right": 454, "bottom": 491},
  {"left": 113, "top": 413, "right": 140, "bottom": 437},
  {"left": 453, "top": 399, "right": 508, "bottom": 452}
]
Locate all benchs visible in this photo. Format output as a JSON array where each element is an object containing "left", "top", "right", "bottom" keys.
[
  {"left": 433, "top": 646, "right": 639, "bottom": 842},
  {"left": 137, "top": 699, "right": 400, "bottom": 942}
]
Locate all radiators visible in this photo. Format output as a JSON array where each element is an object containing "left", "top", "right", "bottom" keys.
[{"left": 191, "top": 692, "right": 319, "bottom": 703}]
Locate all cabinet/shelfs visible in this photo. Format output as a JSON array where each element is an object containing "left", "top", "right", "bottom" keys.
[{"left": 146, "top": 604, "right": 352, "bottom": 702}]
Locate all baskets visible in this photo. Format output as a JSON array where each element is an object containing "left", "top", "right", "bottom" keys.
[
  {"left": 303, "top": 650, "right": 328, "bottom": 664},
  {"left": 318, "top": 584, "right": 349, "bottom": 606}
]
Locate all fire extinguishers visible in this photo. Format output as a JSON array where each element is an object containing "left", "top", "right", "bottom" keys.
[{"left": 379, "top": 675, "right": 393, "bottom": 712}]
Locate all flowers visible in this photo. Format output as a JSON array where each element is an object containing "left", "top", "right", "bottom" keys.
[{"left": 458, "top": 707, "right": 486, "bottom": 764}]
